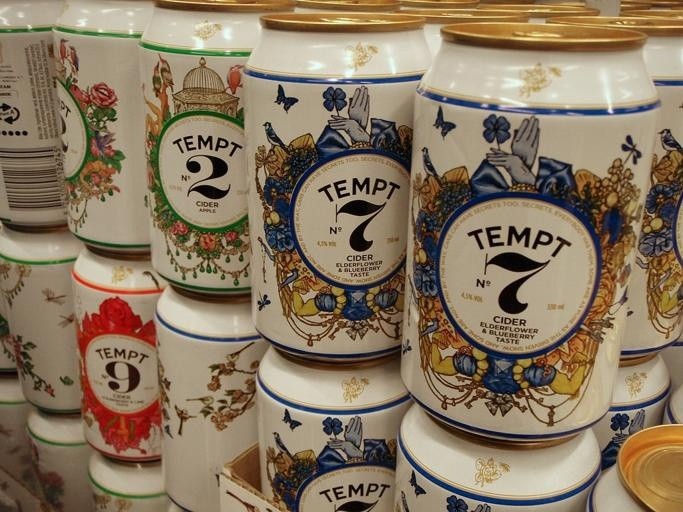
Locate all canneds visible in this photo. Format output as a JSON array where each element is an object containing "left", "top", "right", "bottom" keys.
[
  {"left": 242, "top": 10, "right": 434, "bottom": 362},
  {"left": 587, "top": 425, "right": 682, "bottom": 512},
  {"left": 256, "top": 346, "right": 413, "bottom": 511},
  {"left": 87, "top": 449, "right": 171, "bottom": 511},
  {"left": 154, "top": 284, "right": 270, "bottom": 511},
  {"left": 1, "top": 374, "right": 39, "bottom": 497},
  {"left": 0, "top": 0, "right": 66, "bottom": 233},
  {"left": 393, "top": 403, "right": 602, "bottom": 511},
  {"left": 1, "top": 225, "right": 85, "bottom": 414},
  {"left": 592, "top": 349, "right": 672, "bottom": 471},
  {"left": 392, "top": 8, "right": 531, "bottom": 56},
  {"left": 543, "top": 11, "right": 683, "bottom": 366},
  {"left": 296, "top": 0, "right": 401, "bottom": 14},
  {"left": 70, "top": 246, "right": 169, "bottom": 466},
  {"left": 137, "top": 0, "right": 297, "bottom": 301},
  {"left": 662, "top": 384, "right": 682, "bottom": 424},
  {"left": 399, "top": 22, "right": 661, "bottom": 451},
  {"left": 50, "top": 0, "right": 163, "bottom": 256},
  {"left": 25, "top": 405, "right": 97, "bottom": 512}
]
